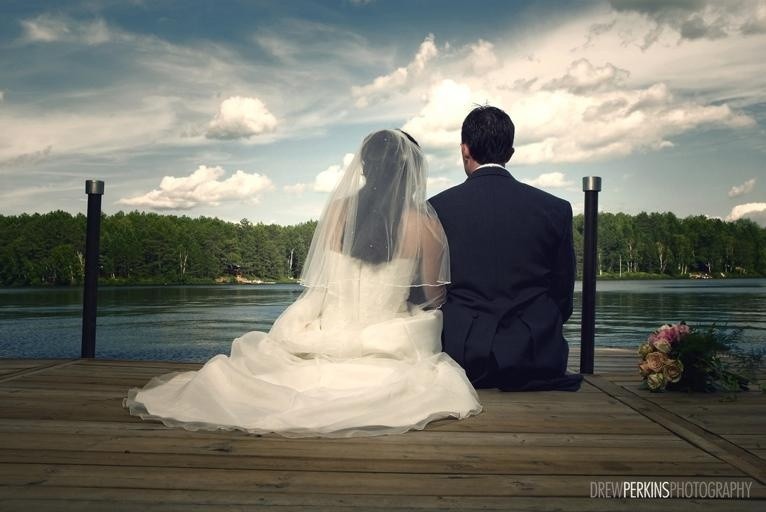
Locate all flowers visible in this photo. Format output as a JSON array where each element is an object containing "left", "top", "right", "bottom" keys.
[{"left": 637, "top": 321, "right": 765, "bottom": 394}]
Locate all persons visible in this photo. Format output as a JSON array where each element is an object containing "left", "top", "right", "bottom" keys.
[
  {"left": 406, "top": 107, "right": 583, "bottom": 392},
  {"left": 122, "top": 127, "right": 484, "bottom": 437}
]
